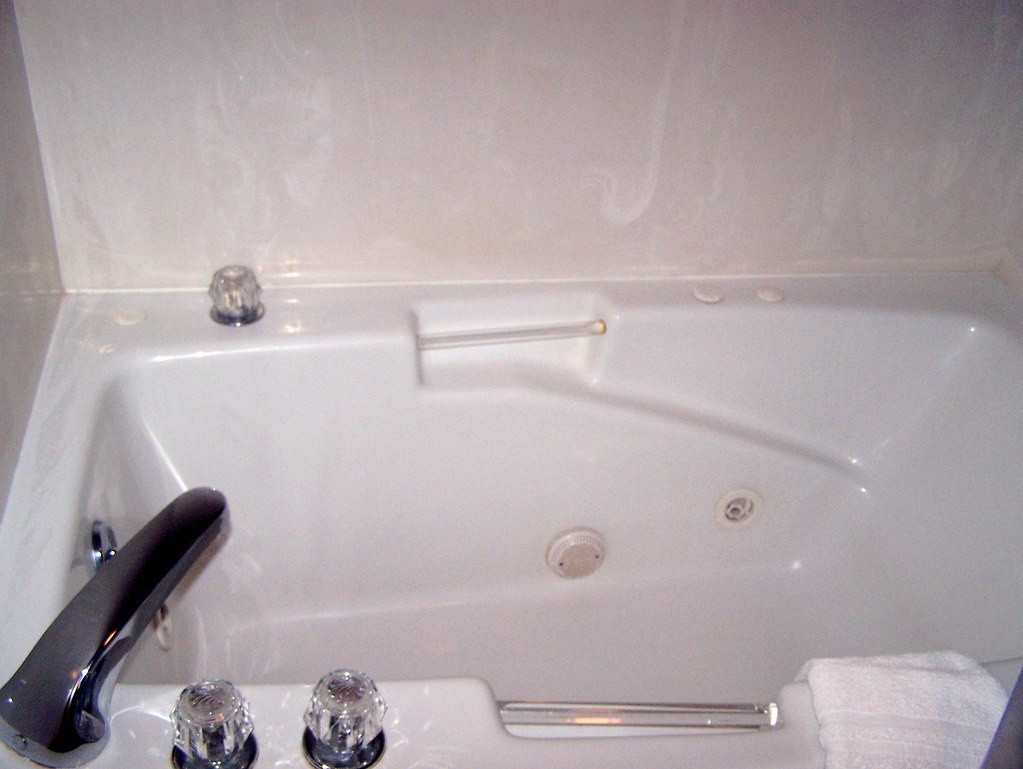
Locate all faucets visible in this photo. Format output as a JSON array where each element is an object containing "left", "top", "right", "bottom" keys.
[{"left": 1, "top": 488, "right": 236, "bottom": 767}]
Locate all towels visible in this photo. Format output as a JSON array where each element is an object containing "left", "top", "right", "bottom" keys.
[{"left": 789, "top": 649, "right": 1010, "bottom": 768}]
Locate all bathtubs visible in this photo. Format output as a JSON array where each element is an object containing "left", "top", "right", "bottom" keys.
[{"left": 0, "top": 269, "right": 1022, "bottom": 768}]
